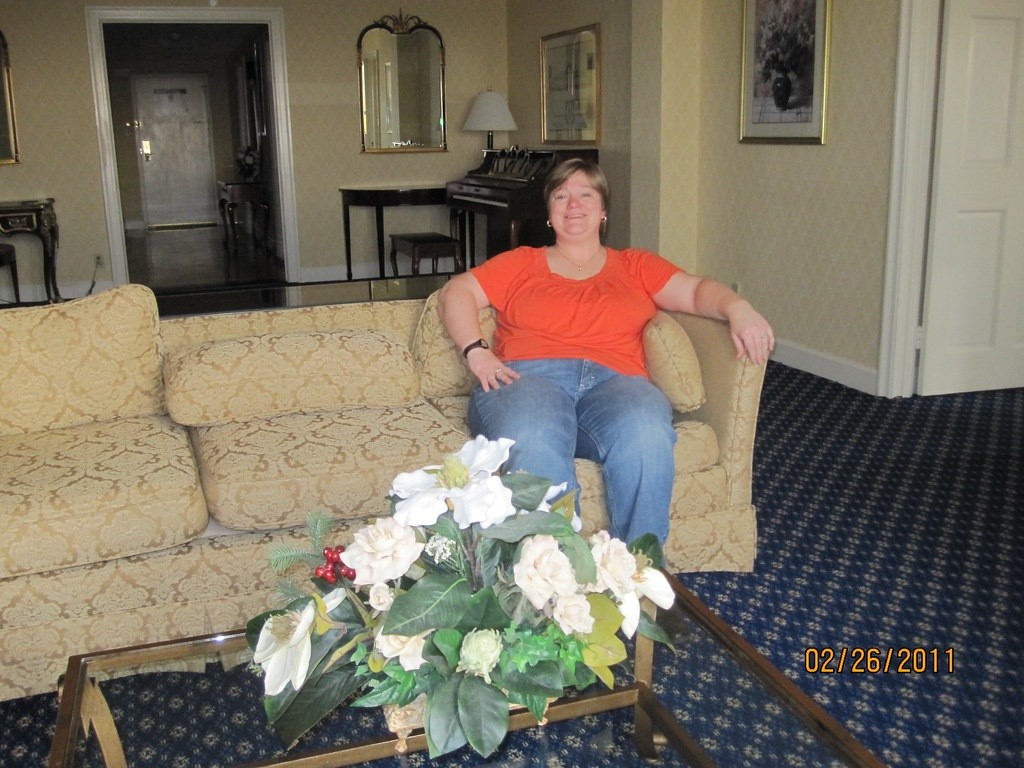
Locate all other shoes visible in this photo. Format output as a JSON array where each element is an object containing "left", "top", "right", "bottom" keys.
[{"left": 655, "top": 606, "right": 690, "bottom": 645}]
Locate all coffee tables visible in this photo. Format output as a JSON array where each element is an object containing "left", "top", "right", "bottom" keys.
[{"left": 40, "top": 568, "right": 889, "bottom": 767}]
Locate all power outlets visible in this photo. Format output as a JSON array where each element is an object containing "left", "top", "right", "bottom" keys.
[{"left": 93, "top": 254, "right": 106, "bottom": 269}]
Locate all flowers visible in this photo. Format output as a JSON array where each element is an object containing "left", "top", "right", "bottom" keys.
[
  {"left": 247, "top": 433, "right": 676, "bottom": 761},
  {"left": 235, "top": 145, "right": 262, "bottom": 168}
]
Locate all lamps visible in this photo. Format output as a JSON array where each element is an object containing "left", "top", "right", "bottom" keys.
[{"left": 462, "top": 87, "right": 521, "bottom": 148}]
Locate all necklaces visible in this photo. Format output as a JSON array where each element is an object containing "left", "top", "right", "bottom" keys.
[{"left": 554, "top": 245, "right": 601, "bottom": 272}]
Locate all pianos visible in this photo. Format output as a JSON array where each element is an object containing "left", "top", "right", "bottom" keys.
[{"left": 446, "top": 143, "right": 600, "bottom": 272}]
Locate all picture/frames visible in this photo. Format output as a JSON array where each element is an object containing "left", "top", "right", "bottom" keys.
[
  {"left": 539, "top": 22, "right": 601, "bottom": 147},
  {"left": 738, "top": 0, "right": 831, "bottom": 147}
]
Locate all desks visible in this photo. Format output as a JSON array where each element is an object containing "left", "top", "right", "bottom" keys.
[
  {"left": 218, "top": 178, "right": 274, "bottom": 258},
  {"left": 0, "top": 200, "right": 61, "bottom": 304},
  {"left": 338, "top": 184, "right": 461, "bottom": 281}
]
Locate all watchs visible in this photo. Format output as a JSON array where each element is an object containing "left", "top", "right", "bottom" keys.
[{"left": 463, "top": 339, "right": 488, "bottom": 358}]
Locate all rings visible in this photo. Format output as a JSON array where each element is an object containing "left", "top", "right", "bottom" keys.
[
  {"left": 762, "top": 336, "right": 766, "bottom": 338},
  {"left": 497, "top": 369, "right": 501, "bottom": 371}
]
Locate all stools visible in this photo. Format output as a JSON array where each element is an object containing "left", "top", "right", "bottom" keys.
[
  {"left": 388, "top": 231, "right": 462, "bottom": 278},
  {"left": 0, "top": 243, "right": 21, "bottom": 304}
]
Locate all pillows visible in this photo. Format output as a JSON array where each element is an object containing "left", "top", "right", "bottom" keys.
[
  {"left": 3, "top": 284, "right": 161, "bottom": 433},
  {"left": 356, "top": 8, "right": 446, "bottom": 153},
  {"left": 642, "top": 313, "right": 707, "bottom": 413},
  {"left": 156, "top": 333, "right": 418, "bottom": 423},
  {"left": 413, "top": 290, "right": 495, "bottom": 394}
]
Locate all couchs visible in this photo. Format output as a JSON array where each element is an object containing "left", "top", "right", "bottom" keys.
[{"left": 2, "top": 283, "right": 770, "bottom": 708}]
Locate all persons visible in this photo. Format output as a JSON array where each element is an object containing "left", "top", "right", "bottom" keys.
[{"left": 436, "top": 158, "right": 776, "bottom": 645}]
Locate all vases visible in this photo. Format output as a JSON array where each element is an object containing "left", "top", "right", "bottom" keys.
[{"left": 241, "top": 164, "right": 255, "bottom": 184}]
